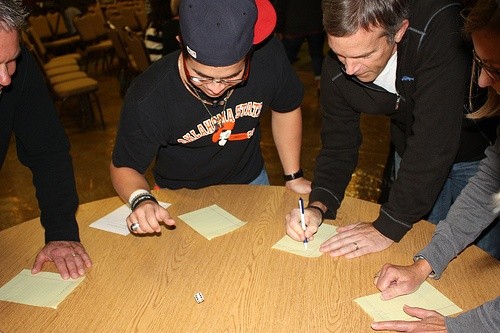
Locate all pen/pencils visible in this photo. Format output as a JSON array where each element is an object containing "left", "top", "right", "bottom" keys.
[{"left": 298, "top": 196, "right": 307, "bottom": 244}]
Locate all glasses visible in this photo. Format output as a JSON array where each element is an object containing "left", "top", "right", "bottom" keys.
[
  {"left": 473, "top": 49, "right": 500, "bottom": 81},
  {"left": 182, "top": 46, "right": 249, "bottom": 86}
]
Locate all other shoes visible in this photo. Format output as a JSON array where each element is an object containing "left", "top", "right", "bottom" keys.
[{"left": 315, "top": 75, "right": 321, "bottom": 80}]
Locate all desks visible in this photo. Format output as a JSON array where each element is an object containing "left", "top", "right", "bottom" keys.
[{"left": 0, "top": 185, "right": 500, "bottom": 333}]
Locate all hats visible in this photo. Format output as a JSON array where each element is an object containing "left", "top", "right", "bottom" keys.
[{"left": 178, "top": 0, "right": 277, "bottom": 67}]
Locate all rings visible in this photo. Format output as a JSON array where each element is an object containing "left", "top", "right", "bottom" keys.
[
  {"left": 131, "top": 224, "right": 139, "bottom": 230},
  {"left": 353, "top": 242, "right": 358, "bottom": 249},
  {"left": 73, "top": 253, "right": 78, "bottom": 256},
  {"left": 374, "top": 274, "right": 380, "bottom": 276}
]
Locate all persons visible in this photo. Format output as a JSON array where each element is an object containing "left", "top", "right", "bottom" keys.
[
  {"left": 282, "top": 0, "right": 323, "bottom": 97},
  {"left": 0, "top": 0, "right": 95, "bottom": 280},
  {"left": 145, "top": 0, "right": 177, "bottom": 61},
  {"left": 371, "top": 0, "right": 500, "bottom": 333},
  {"left": 285, "top": 0, "right": 500, "bottom": 262},
  {"left": 111, "top": 0, "right": 310, "bottom": 234}
]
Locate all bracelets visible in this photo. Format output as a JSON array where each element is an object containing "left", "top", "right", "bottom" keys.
[
  {"left": 308, "top": 205, "right": 324, "bottom": 227},
  {"left": 131, "top": 193, "right": 158, "bottom": 210},
  {"left": 129, "top": 189, "right": 149, "bottom": 204}
]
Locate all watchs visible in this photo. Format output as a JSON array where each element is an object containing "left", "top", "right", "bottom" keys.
[{"left": 284, "top": 169, "right": 303, "bottom": 181}]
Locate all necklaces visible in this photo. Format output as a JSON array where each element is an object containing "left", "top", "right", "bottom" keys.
[{"left": 178, "top": 53, "right": 231, "bottom": 128}]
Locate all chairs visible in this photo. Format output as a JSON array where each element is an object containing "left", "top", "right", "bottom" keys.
[{"left": 20, "top": 0, "right": 181, "bottom": 134}]
